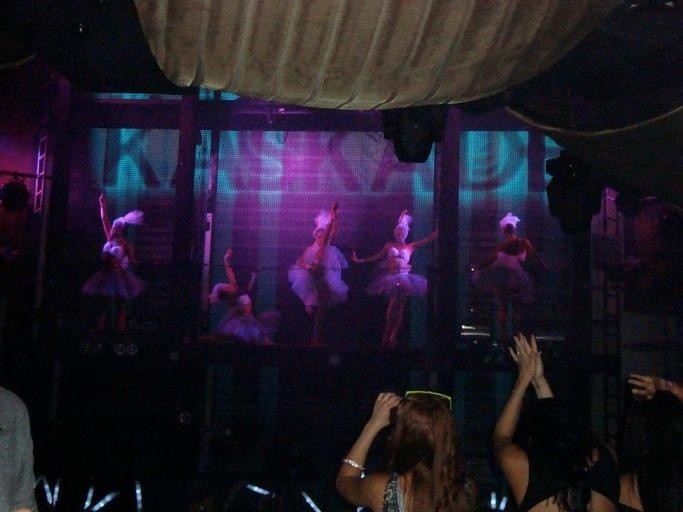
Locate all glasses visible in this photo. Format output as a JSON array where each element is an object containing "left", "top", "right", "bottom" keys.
[{"left": 404, "top": 390, "right": 453, "bottom": 412}]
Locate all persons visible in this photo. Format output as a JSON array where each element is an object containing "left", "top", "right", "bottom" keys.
[
  {"left": 464, "top": 209, "right": 555, "bottom": 336},
  {"left": 0, "top": 325, "right": 683, "bottom": 510},
  {"left": 81, "top": 189, "right": 148, "bottom": 327},
  {"left": 350, "top": 208, "right": 439, "bottom": 348},
  {"left": 285, "top": 201, "right": 352, "bottom": 346},
  {"left": 209, "top": 246, "right": 280, "bottom": 345}
]
